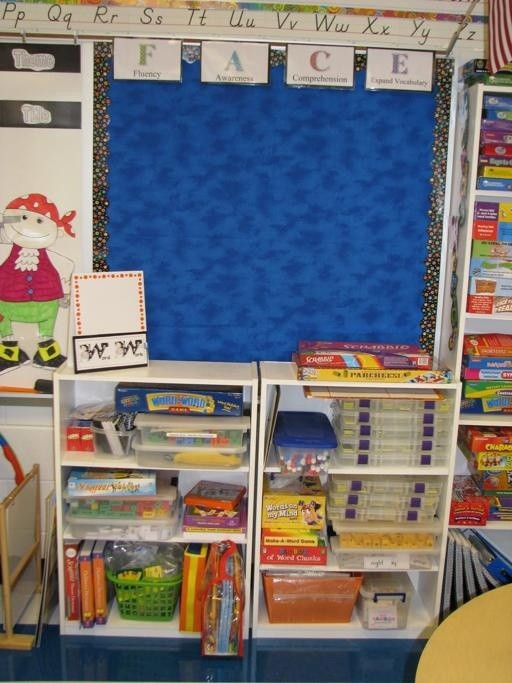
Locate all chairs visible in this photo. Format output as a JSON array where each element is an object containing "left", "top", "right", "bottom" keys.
[{"left": 411, "top": 585, "right": 512, "bottom": 683}]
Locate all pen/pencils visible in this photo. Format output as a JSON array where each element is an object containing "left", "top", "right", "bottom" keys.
[{"left": 278, "top": 448, "right": 332, "bottom": 477}]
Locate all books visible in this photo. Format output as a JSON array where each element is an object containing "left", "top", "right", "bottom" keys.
[
  {"left": 438, "top": 526, "right": 511, "bottom": 627},
  {"left": 62, "top": 539, "right": 118, "bottom": 628}
]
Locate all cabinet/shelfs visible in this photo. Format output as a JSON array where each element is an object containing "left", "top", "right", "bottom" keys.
[
  {"left": 447, "top": 85, "right": 511, "bottom": 530},
  {"left": 51, "top": 357, "right": 258, "bottom": 639},
  {"left": 256, "top": 355, "right": 464, "bottom": 644}
]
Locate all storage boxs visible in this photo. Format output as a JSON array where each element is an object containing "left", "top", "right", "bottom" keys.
[
  {"left": 64, "top": 382, "right": 254, "bottom": 541},
  {"left": 325, "top": 394, "right": 451, "bottom": 571},
  {"left": 359, "top": 571, "right": 413, "bottom": 629},
  {"left": 447, "top": 473, "right": 488, "bottom": 526},
  {"left": 260, "top": 569, "right": 364, "bottom": 626},
  {"left": 259, "top": 471, "right": 328, "bottom": 531},
  {"left": 271, "top": 405, "right": 338, "bottom": 476}
]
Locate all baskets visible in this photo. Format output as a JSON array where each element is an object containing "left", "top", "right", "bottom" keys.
[{"left": 108, "top": 569, "right": 180, "bottom": 622}]
